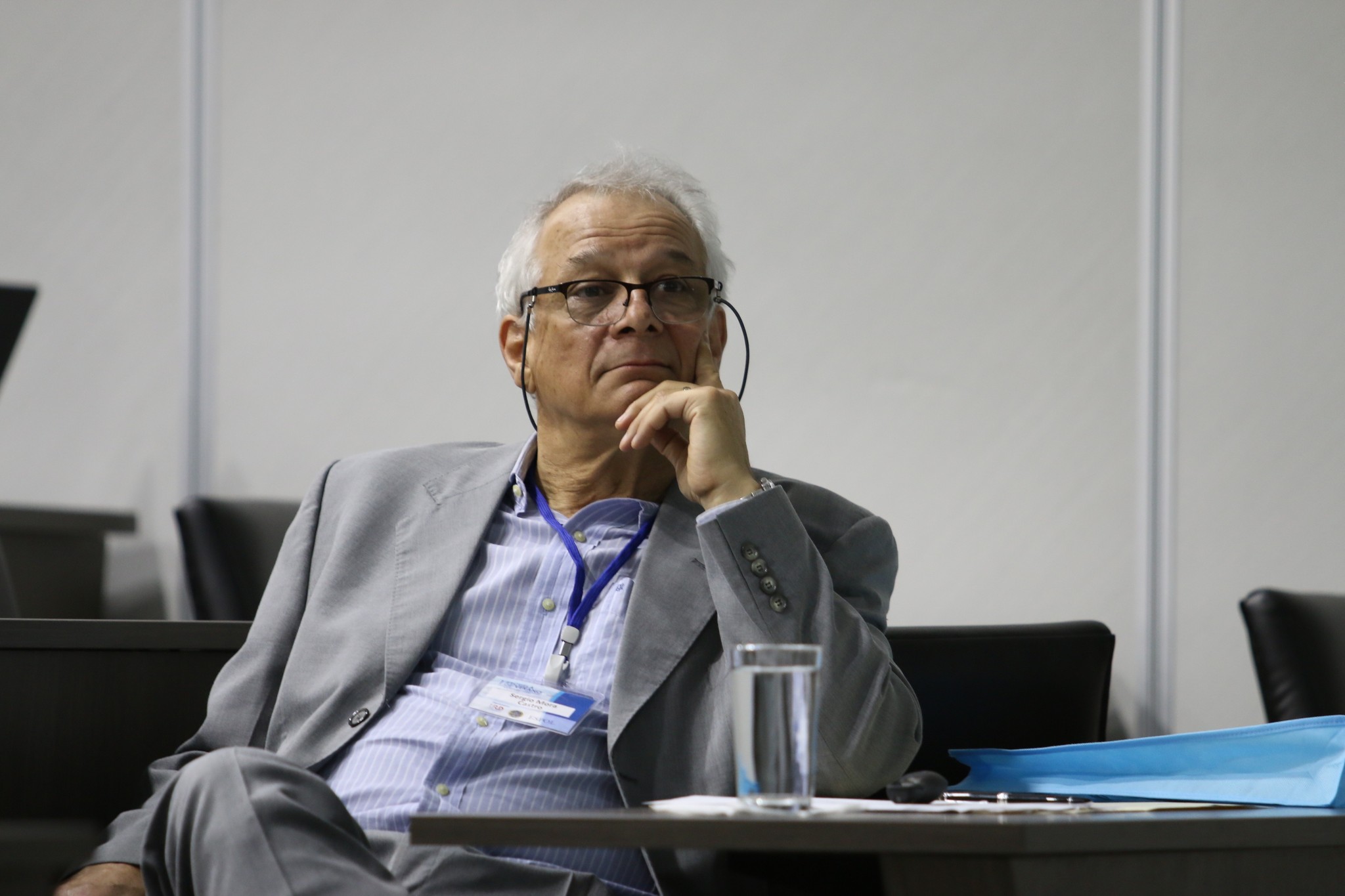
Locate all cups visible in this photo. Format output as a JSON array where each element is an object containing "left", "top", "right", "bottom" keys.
[{"left": 729, "top": 643, "right": 822, "bottom": 810}]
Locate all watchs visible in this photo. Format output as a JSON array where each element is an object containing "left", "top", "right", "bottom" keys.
[{"left": 734, "top": 478, "right": 776, "bottom": 499}]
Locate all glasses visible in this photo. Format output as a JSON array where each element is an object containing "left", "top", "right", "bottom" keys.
[{"left": 519, "top": 277, "right": 722, "bottom": 326}]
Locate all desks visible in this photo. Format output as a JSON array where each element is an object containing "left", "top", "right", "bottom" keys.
[{"left": 407, "top": 796, "right": 1344, "bottom": 895}]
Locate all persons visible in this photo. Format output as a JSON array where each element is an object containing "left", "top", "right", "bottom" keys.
[{"left": 58, "top": 154, "right": 920, "bottom": 896}]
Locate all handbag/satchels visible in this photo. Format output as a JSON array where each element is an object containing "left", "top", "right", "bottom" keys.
[{"left": 940, "top": 712, "right": 1345, "bottom": 820}]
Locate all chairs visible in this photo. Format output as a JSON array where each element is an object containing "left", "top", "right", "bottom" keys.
[
  {"left": 177, "top": 497, "right": 302, "bottom": 619},
  {"left": 1240, "top": 591, "right": 1345, "bottom": 723},
  {"left": 884, "top": 619, "right": 1115, "bottom": 785}
]
